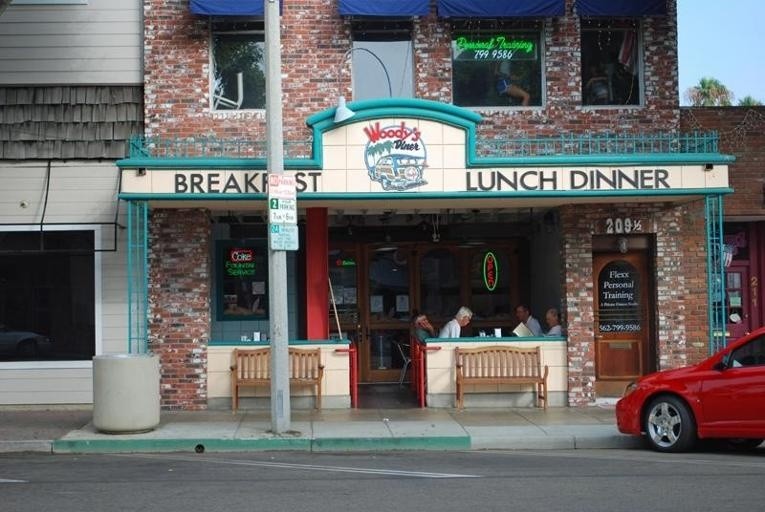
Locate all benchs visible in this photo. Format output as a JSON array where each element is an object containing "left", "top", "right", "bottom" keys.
[
  {"left": 230, "top": 346, "right": 323, "bottom": 414},
  {"left": 456, "top": 346, "right": 549, "bottom": 411}
]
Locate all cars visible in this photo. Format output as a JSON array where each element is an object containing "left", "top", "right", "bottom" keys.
[{"left": 616, "top": 327, "right": 765, "bottom": 453}]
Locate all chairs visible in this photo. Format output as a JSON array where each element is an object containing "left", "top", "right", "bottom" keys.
[{"left": 393, "top": 338, "right": 411, "bottom": 385}]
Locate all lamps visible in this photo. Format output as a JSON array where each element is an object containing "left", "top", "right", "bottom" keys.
[
  {"left": 334, "top": 47, "right": 391, "bottom": 123},
  {"left": 617, "top": 236, "right": 629, "bottom": 254}
]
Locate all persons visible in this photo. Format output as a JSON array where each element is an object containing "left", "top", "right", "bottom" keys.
[
  {"left": 494, "top": 60, "right": 531, "bottom": 106},
  {"left": 413, "top": 313, "right": 436, "bottom": 346},
  {"left": 516, "top": 303, "right": 542, "bottom": 336},
  {"left": 545, "top": 308, "right": 562, "bottom": 337},
  {"left": 438, "top": 306, "right": 474, "bottom": 338}
]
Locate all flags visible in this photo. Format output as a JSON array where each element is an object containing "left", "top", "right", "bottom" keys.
[{"left": 717, "top": 242, "right": 735, "bottom": 269}]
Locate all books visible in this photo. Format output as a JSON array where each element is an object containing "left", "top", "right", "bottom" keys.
[{"left": 511, "top": 322, "right": 534, "bottom": 336}]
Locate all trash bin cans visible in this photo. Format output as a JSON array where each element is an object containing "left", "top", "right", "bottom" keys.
[{"left": 92, "top": 354, "right": 161, "bottom": 435}]
